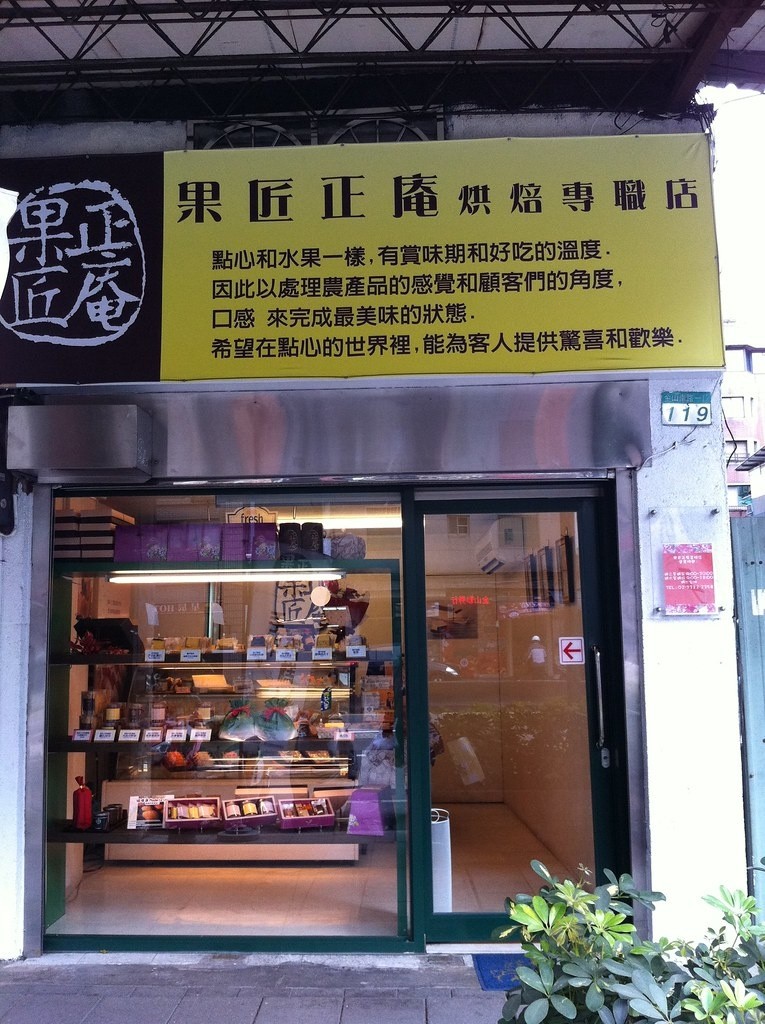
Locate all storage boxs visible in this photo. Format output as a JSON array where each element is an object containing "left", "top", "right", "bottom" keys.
[
  {"left": 112, "top": 523, "right": 166, "bottom": 562},
  {"left": 221, "top": 795, "right": 278, "bottom": 830},
  {"left": 54, "top": 507, "right": 135, "bottom": 560},
  {"left": 278, "top": 797, "right": 335, "bottom": 829},
  {"left": 168, "top": 522, "right": 221, "bottom": 561},
  {"left": 164, "top": 797, "right": 220, "bottom": 830},
  {"left": 221, "top": 522, "right": 280, "bottom": 560}
]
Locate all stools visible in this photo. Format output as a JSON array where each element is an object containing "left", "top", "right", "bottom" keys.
[{"left": 347, "top": 784, "right": 397, "bottom": 837}]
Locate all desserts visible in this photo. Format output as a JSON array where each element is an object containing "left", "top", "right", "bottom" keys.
[{"left": 150, "top": 635, "right": 363, "bottom": 650}]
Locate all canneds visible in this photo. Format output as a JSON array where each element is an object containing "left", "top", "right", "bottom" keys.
[
  {"left": 240, "top": 800, "right": 258, "bottom": 815},
  {"left": 80, "top": 691, "right": 216, "bottom": 730},
  {"left": 257, "top": 799, "right": 274, "bottom": 814},
  {"left": 226, "top": 802, "right": 241, "bottom": 818}
]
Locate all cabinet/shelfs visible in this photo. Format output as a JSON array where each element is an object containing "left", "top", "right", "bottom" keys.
[{"left": 45, "top": 559, "right": 406, "bottom": 861}]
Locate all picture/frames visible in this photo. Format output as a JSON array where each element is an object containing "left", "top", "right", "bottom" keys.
[
  {"left": 537, "top": 545, "right": 553, "bottom": 608},
  {"left": 523, "top": 554, "right": 538, "bottom": 609},
  {"left": 554, "top": 535, "right": 574, "bottom": 604}
]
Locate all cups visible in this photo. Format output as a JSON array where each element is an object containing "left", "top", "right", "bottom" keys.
[{"left": 94, "top": 803, "right": 123, "bottom": 830}]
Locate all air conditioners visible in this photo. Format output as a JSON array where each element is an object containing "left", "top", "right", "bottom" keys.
[{"left": 474, "top": 515, "right": 524, "bottom": 575}]
